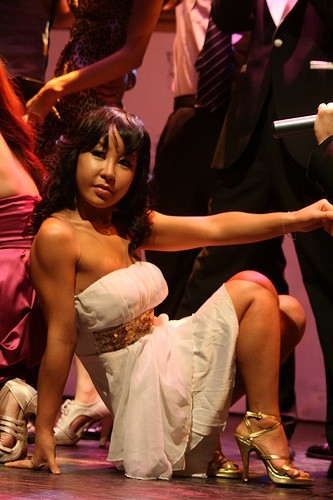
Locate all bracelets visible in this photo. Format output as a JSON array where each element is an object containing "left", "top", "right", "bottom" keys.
[{"left": 282, "top": 210, "right": 298, "bottom": 240}]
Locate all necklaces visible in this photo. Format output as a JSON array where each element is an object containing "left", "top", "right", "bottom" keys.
[{"left": 77, "top": 206, "right": 117, "bottom": 237}]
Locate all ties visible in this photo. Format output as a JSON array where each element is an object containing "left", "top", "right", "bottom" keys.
[{"left": 194, "top": 11, "right": 233, "bottom": 115}]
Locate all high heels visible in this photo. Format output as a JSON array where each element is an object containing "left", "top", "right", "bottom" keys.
[
  {"left": 208, "top": 451, "right": 244, "bottom": 479},
  {"left": 53, "top": 399, "right": 114, "bottom": 447},
  {"left": 234, "top": 410, "right": 315, "bottom": 487},
  {"left": 0, "top": 378, "right": 38, "bottom": 465}
]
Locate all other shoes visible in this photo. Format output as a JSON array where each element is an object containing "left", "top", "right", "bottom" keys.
[{"left": 306, "top": 443, "right": 332, "bottom": 460}]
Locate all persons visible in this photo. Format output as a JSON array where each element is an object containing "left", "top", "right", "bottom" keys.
[
  {"left": 26, "top": 0, "right": 164, "bottom": 447},
  {"left": 146, "top": 0, "right": 239, "bottom": 320},
  {"left": 206, "top": 0, "right": 333, "bottom": 483},
  {"left": 1, "top": 106, "right": 333, "bottom": 486},
  {"left": 0, "top": 0, "right": 60, "bottom": 105},
  {"left": 0, "top": 58, "right": 52, "bottom": 464}
]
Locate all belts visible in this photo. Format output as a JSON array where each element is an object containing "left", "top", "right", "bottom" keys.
[{"left": 173, "top": 94, "right": 194, "bottom": 112}]
